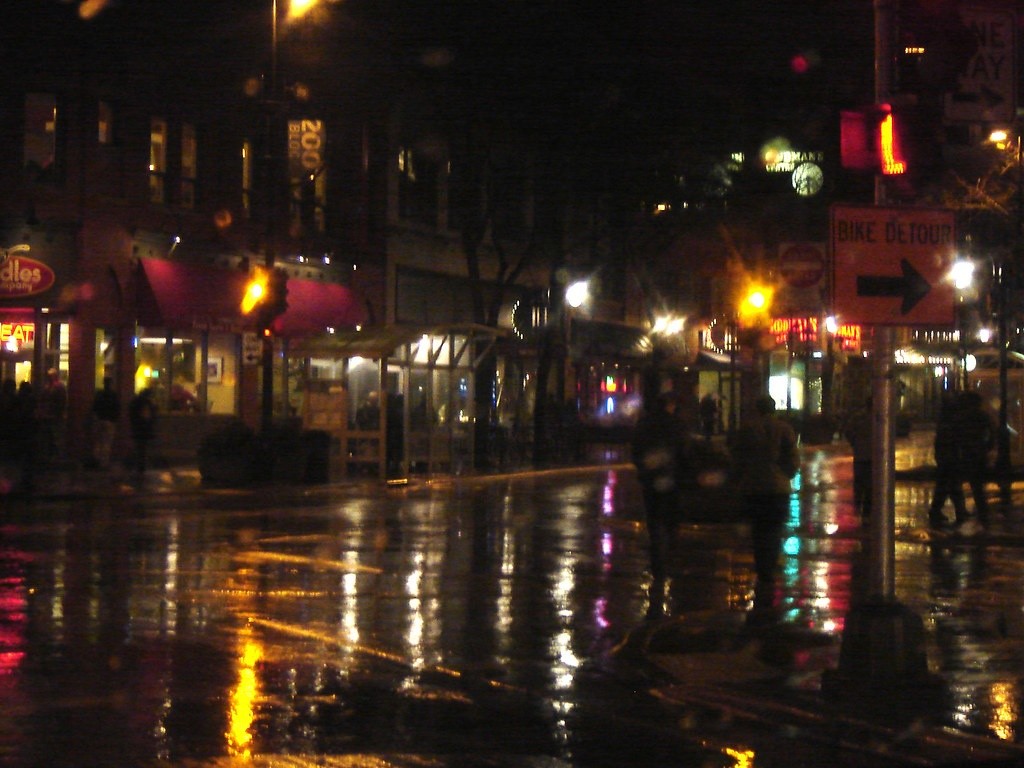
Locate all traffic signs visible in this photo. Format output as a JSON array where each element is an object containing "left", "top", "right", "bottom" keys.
[{"left": 831, "top": 203, "right": 956, "bottom": 325}]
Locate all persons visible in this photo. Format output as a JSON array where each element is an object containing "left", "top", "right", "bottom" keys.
[
  {"left": 841, "top": 397, "right": 875, "bottom": 524},
  {"left": 632, "top": 342, "right": 698, "bottom": 560},
  {"left": 737, "top": 394, "right": 802, "bottom": 571},
  {"left": 927, "top": 389, "right": 984, "bottom": 524},
  {"left": 950, "top": 393, "right": 998, "bottom": 519},
  {"left": 0, "top": 335, "right": 502, "bottom": 485},
  {"left": 699, "top": 393, "right": 719, "bottom": 438}
]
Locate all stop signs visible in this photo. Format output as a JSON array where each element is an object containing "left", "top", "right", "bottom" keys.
[{"left": 779, "top": 243, "right": 826, "bottom": 288}]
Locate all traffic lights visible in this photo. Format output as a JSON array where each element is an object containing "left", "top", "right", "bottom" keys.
[{"left": 864, "top": 100, "right": 924, "bottom": 180}]
